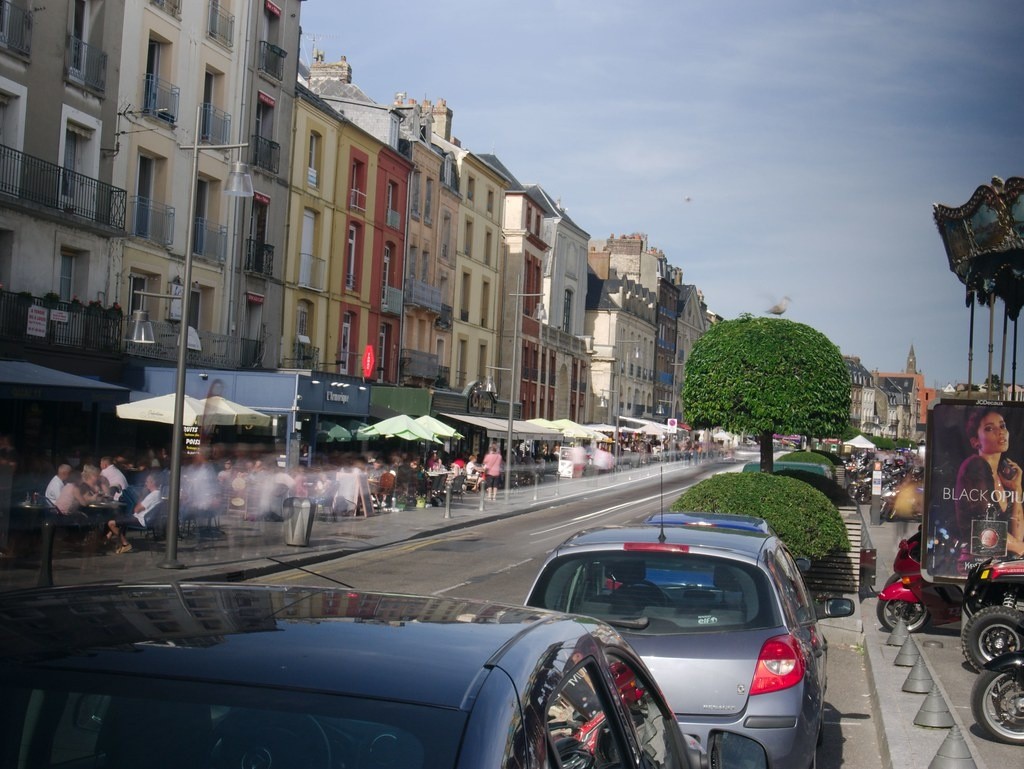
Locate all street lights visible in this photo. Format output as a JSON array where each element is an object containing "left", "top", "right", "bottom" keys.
[
  {"left": 159, "top": 103, "right": 256, "bottom": 568},
  {"left": 503, "top": 273, "right": 549, "bottom": 506},
  {"left": 615, "top": 324, "right": 642, "bottom": 470}
]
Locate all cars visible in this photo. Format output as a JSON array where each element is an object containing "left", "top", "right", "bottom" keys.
[
  {"left": 523, "top": 526, "right": 855, "bottom": 769},
  {"left": 0, "top": 580, "right": 695, "bottom": 769},
  {"left": 647, "top": 510, "right": 810, "bottom": 573},
  {"left": 960, "top": 554, "right": 1024, "bottom": 672}
]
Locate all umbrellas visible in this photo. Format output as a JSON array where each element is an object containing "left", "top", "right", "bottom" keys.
[
  {"left": 526, "top": 417, "right": 664, "bottom": 441},
  {"left": 843, "top": 435, "right": 877, "bottom": 448},
  {"left": 115, "top": 389, "right": 270, "bottom": 435},
  {"left": 313, "top": 414, "right": 465, "bottom": 446},
  {"left": 714, "top": 432, "right": 731, "bottom": 440}
]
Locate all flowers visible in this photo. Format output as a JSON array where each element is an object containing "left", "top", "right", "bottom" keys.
[
  {"left": 70, "top": 294, "right": 84, "bottom": 313},
  {"left": 111, "top": 301, "right": 126, "bottom": 321},
  {"left": 88, "top": 300, "right": 103, "bottom": 310}
]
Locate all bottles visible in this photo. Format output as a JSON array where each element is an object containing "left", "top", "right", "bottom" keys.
[{"left": 969, "top": 502, "right": 1009, "bottom": 559}]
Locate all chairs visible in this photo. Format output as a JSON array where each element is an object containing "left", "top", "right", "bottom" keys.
[{"left": 59, "top": 456, "right": 478, "bottom": 553}]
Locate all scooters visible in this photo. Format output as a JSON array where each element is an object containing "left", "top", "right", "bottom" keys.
[
  {"left": 843, "top": 450, "right": 925, "bottom": 522},
  {"left": 970, "top": 651, "right": 1024, "bottom": 746}
]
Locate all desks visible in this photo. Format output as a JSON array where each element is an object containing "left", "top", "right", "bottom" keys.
[
  {"left": 16, "top": 501, "right": 57, "bottom": 568},
  {"left": 86, "top": 500, "right": 127, "bottom": 556}
]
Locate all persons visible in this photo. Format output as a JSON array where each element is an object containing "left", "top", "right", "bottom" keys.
[
  {"left": 512, "top": 439, "right": 560, "bottom": 484},
  {"left": 0, "top": 435, "right": 506, "bottom": 555},
  {"left": 955, "top": 409, "right": 1024, "bottom": 576}
]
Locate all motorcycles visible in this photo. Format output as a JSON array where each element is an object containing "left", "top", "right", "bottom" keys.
[{"left": 870, "top": 526, "right": 961, "bottom": 639}]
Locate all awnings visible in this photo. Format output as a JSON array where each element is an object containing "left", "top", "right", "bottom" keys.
[
  {"left": 0, "top": 358, "right": 130, "bottom": 402},
  {"left": 442, "top": 411, "right": 562, "bottom": 440}
]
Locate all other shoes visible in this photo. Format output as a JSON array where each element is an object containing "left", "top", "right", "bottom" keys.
[{"left": 116, "top": 544, "right": 133, "bottom": 553}]
